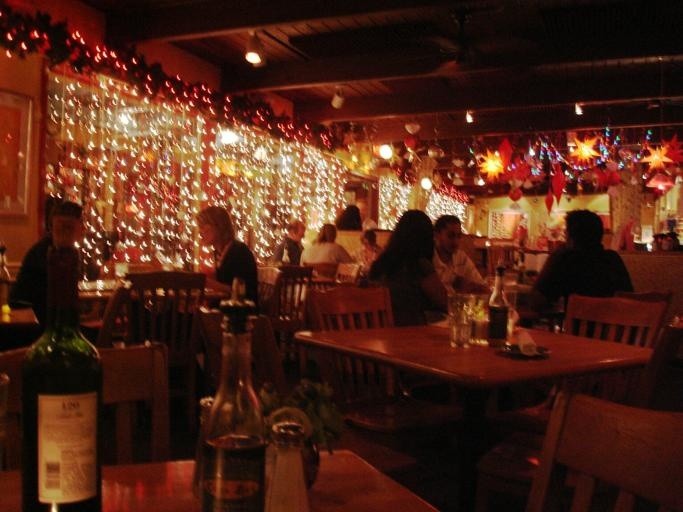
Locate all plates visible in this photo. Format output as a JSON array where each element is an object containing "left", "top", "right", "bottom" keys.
[{"left": 505, "top": 345, "right": 552, "bottom": 359}]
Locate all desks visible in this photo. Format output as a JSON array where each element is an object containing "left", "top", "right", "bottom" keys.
[
  {"left": 293, "top": 326, "right": 655, "bottom": 512},
  {"left": 100, "top": 450, "right": 438, "bottom": 510},
  {"left": 0, "top": 470, "right": 24, "bottom": 510}
]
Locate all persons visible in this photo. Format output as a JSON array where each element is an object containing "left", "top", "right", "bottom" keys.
[
  {"left": 518, "top": 209, "right": 634, "bottom": 338},
  {"left": 360, "top": 209, "right": 449, "bottom": 403},
  {"left": 653, "top": 218, "right": 680, "bottom": 253},
  {"left": 270, "top": 218, "right": 304, "bottom": 266},
  {"left": 8, "top": 209, "right": 100, "bottom": 341},
  {"left": 358, "top": 205, "right": 379, "bottom": 230},
  {"left": 294, "top": 223, "right": 356, "bottom": 319},
  {"left": 195, "top": 205, "right": 261, "bottom": 335},
  {"left": 350, "top": 231, "right": 383, "bottom": 283},
  {"left": 431, "top": 214, "right": 486, "bottom": 297},
  {"left": 617, "top": 215, "right": 647, "bottom": 251},
  {"left": 517, "top": 213, "right": 529, "bottom": 262}
]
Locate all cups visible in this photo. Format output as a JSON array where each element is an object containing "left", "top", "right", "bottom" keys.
[
  {"left": 448, "top": 293, "right": 472, "bottom": 349},
  {"left": 504, "top": 288, "right": 516, "bottom": 319}
]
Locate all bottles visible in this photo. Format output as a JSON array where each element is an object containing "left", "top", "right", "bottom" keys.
[
  {"left": 0, "top": 237, "right": 8, "bottom": 316},
  {"left": 268, "top": 422, "right": 312, "bottom": 511},
  {"left": 487, "top": 265, "right": 509, "bottom": 348},
  {"left": 19, "top": 256, "right": 105, "bottom": 512},
  {"left": 197, "top": 276, "right": 266, "bottom": 508},
  {"left": 472, "top": 298, "right": 488, "bottom": 345}
]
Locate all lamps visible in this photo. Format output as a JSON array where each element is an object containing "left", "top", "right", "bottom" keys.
[
  {"left": 328, "top": 84, "right": 352, "bottom": 108},
  {"left": 240, "top": 28, "right": 262, "bottom": 65}
]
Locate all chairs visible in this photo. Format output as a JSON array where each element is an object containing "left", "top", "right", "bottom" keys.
[
  {"left": 253, "top": 262, "right": 460, "bottom": 476},
  {"left": 561, "top": 289, "right": 683, "bottom": 405},
  {"left": 527, "top": 389, "right": 682, "bottom": 511},
  {"left": 479, "top": 241, "right": 547, "bottom": 274},
  {"left": 3, "top": 272, "right": 207, "bottom": 470}
]
